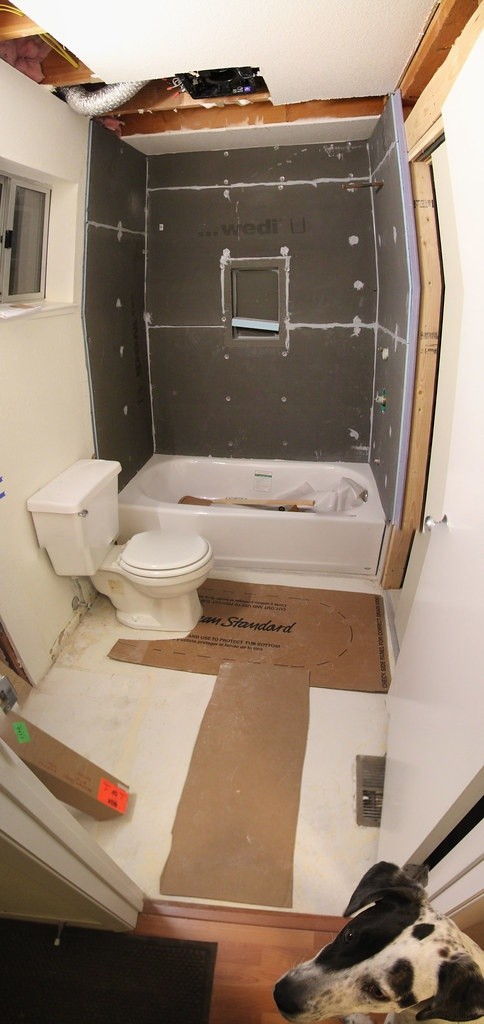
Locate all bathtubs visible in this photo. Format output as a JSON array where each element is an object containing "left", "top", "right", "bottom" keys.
[{"left": 117, "top": 454, "right": 386, "bottom": 578}]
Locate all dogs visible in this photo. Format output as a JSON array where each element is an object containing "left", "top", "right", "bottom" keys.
[{"left": 272, "top": 861, "right": 484, "bottom": 1024}]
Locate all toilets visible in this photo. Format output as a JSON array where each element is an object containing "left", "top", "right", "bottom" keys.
[{"left": 26, "top": 457, "right": 217, "bottom": 633}]
